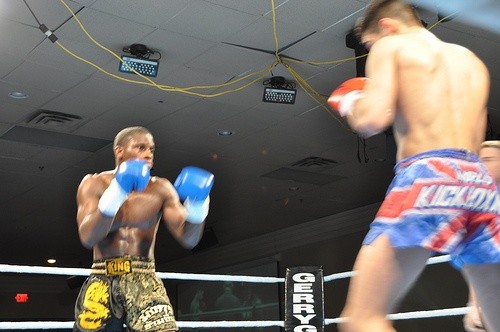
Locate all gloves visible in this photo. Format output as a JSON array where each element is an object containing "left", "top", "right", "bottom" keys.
[
  {"left": 97, "top": 157, "right": 150, "bottom": 219},
  {"left": 173, "top": 165, "right": 215, "bottom": 225},
  {"left": 328, "top": 77, "right": 368, "bottom": 119}
]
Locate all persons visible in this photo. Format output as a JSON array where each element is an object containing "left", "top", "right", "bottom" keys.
[
  {"left": 328, "top": 0, "right": 500, "bottom": 332},
  {"left": 73, "top": 126, "right": 213, "bottom": 332},
  {"left": 463, "top": 141, "right": 500, "bottom": 332}
]
